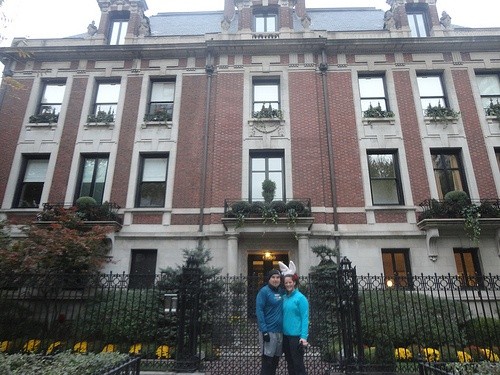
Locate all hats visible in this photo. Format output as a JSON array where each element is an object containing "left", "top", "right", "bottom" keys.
[{"left": 264, "top": 269, "right": 280, "bottom": 283}]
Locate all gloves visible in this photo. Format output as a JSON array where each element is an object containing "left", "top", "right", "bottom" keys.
[{"left": 262, "top": 331, "right": 270, "bottom": 342}]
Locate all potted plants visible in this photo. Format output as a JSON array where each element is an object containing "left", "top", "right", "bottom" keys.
[{"left": 249, "top": 201, "right": 286, "bottom": 218}]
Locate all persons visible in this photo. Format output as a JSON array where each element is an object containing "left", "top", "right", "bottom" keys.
[
  {"left": 256, "top": 269, "right": 284, "bottom": 375},
  {"left": 278, "top": 261, "right": 309, "bottom": 375}
]
